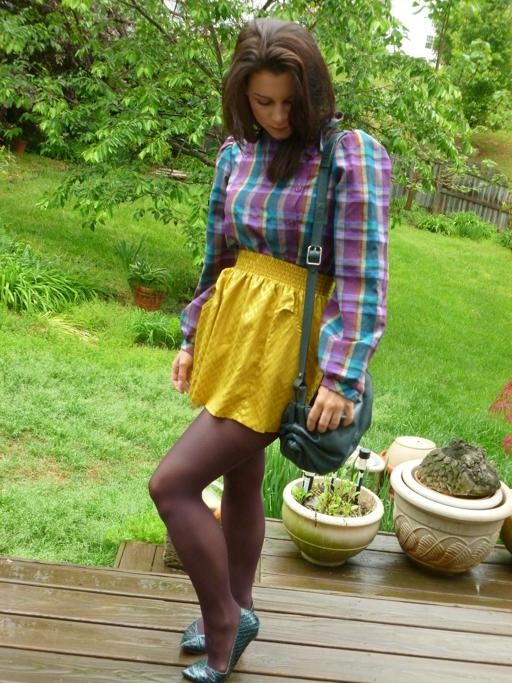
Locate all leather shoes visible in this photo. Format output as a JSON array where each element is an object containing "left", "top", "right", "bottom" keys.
[
  {"left": 180, "top": 600, "right": 254, "bottom": 654},
  {"left": 181, "top": 607, "right": 259, "bottom": 683}
]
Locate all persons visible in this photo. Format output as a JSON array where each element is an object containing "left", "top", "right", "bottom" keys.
[{"left": 148, "top": 22, "right": 390, "bottom": 682}]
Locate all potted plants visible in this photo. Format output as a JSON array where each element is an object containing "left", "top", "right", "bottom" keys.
[
  {"left": 281, "top": 459, "right": 385, "bottom": 568},
  {"left": 128, "top": 259, "right": 174, "bottom": 312}
]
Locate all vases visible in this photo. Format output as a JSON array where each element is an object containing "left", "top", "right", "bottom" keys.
[
  {"left": 379, "top": 436, "right": 438, "bottom": 500},
  {"left": 389, "top": 457, "right": 512, "bottom": 576},
  {"left": 8, "top": 139, "right": 29, "bottom": 153},
  {"left": 500, "top": 515, "right": 512, "bottom": 553},
  {"left": 163, "top": 480, "right": 225, "bottom": 571}
]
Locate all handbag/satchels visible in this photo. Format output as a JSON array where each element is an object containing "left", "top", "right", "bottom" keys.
[{"left": 280, "top": 369, "right": 373, "bottom": 475}]
